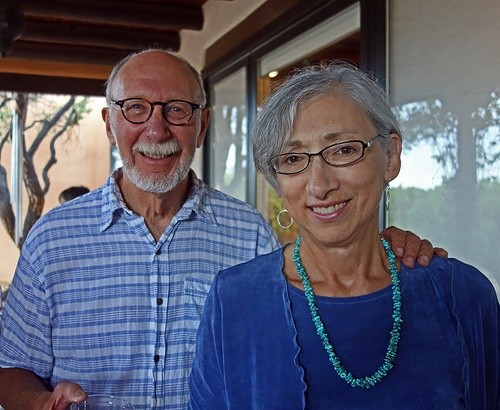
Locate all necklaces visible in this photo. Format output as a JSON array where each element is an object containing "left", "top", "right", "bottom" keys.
[{"left": 292, "top": 233, "right": 403, "bottom": 388}]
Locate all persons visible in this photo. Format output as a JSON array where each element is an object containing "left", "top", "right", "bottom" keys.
[
  {"left": 0, "top": 49, "right": 450, "bottom": 410},
  {"left": 187, "top": 62, "right": 499, "bottom": 410},
  {"left": 58, "top": 186, "right": 90, "bottom": 206}
]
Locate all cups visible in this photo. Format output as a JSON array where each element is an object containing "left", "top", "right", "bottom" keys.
[{"left": 70, "top": 394, "right": 135, "bottom": 410}]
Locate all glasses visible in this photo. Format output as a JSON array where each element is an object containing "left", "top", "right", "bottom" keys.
[
  {"left": 110, "top": 97, "right": 206, "bottom": 126},
  {"left": 270, "top": 134, "right": 381, "bottom": 175}
]
